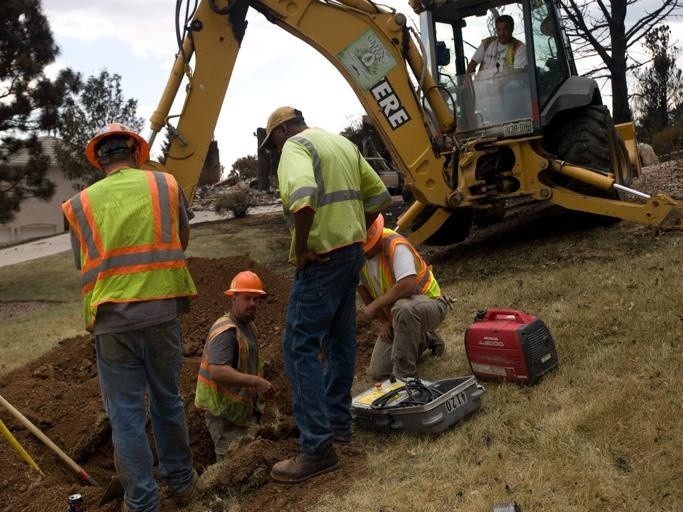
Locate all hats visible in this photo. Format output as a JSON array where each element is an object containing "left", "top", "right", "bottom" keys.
[{"left": 259, "top": 106, "right": 304, "bottom": 149}]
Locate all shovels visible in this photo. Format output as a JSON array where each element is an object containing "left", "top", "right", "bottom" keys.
[{"left": 94, "top": 470, "right": 127, "bottom": 508}]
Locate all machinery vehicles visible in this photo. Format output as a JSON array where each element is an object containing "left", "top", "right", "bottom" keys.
[{"left": 146, "top": 1, "right": 682, "bottom": 250}]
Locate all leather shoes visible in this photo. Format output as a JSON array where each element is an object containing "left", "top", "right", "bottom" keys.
[
  {"left": 270, "top": 448, "right": 339, "bottom": 482},
  {"left": 174, "top": 469, "right": 198, "bottom": 503},
  {"left": 289, "top": 425, "right": 352, "bottom": 443},
  {"left": 428, "top": 330, "right": 444, "bottom": 357}
]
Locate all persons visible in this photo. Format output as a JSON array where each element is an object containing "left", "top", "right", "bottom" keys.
[
  {"left": 353, "top": 210, "right": 453, "bottom": 387},
  {"left": 256, "top": 102, "right": 397, "bottom": 484},
  {"left": 466, "top": 16, "right": 530, "bottom": 75},
  {"left": 62, "top": 119, "right": 206, "bottom": 511},
  {"left": 191, "top": 270, "right": 279, "bottom": 457}
]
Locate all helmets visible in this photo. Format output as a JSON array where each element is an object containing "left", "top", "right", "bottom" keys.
[
  {"left": 363, "top": 212, "right": 384, "bottom": 253},
  {"left": 223, "top": 270, "right": 267, "bottom": 296},
  {"left": 86, "top": 123, "right": 149, "bottom": 168}
]
